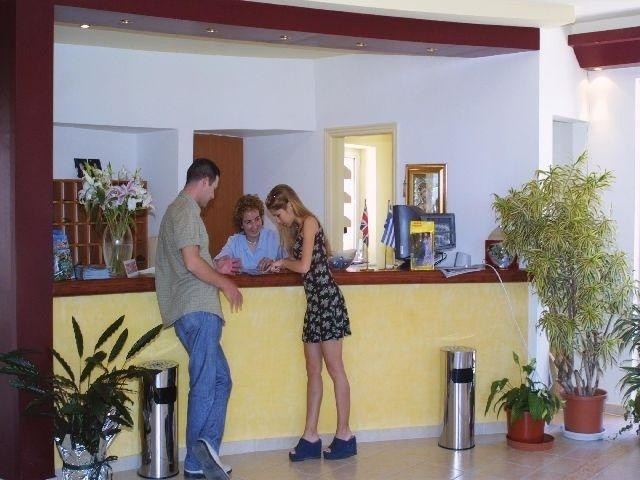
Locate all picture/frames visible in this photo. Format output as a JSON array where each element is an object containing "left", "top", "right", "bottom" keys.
[{"left": 406, "top": 163, "right": 446, "bottom": 212}]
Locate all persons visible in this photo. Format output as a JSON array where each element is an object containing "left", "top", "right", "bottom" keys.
[
  {"left": 212, "top": 193, "right": 290, "bottom": 272},
  {"left": 416, "top": 182, "right": 436, "bottom": 214},
  {"left": 264, "top": 184, "right": 357, "bottom": 462},
  {"left": 152, "top": 158, "right": 244, "bottom": 480}
]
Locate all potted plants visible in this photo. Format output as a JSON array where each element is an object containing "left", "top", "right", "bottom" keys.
[
  {"left": 0, "top": 314, "right": 164, "bottom": 480},
  {"left": 484, "top": 351, "right": 566, "bottom": 451},
  {"left": 490, "top": 147, "right": 640, "bottom": 442}
]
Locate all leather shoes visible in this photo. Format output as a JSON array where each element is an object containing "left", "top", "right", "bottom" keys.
[
  {"left": 184, "top": 464, "right": 232, "bottom": 479},
  {"left": 192, "top": 439, "right": 231, "bottom": 479}
]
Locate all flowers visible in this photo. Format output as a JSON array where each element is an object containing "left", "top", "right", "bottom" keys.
[{"left": 71, "top": 161, "right": 156, "bottom": 275}]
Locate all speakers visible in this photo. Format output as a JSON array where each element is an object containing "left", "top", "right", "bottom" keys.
[{"left": 454, "top": 251, "right": 471, "bottom": 269}]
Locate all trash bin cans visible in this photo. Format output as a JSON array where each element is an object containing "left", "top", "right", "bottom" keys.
[
  {"left": 137, "top": 359, "right": 179, "bottom": 479},
  {"left": 438, "top": 346, "right": 476, "bottom": 450}
]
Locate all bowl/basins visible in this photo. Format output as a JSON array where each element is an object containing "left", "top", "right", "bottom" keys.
[{"left": 327, "top": 248, "right": 357, "bottom": 271}]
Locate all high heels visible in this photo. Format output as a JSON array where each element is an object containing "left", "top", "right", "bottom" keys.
[
  {"left": 289, "top": 437, "right": 322, "bottom": 462},
  {"left": 323, "top": 435, "right": 358, "bottom": 461}
]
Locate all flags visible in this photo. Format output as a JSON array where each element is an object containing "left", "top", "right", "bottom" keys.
[
  {"left": 359, "top": 200, "right": 369, "bottom": 248},
  {"left": 379, "top": 208, "right": 396, "bottom": 252}
]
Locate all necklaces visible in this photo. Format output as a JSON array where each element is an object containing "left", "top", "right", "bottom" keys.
[{"left": 246, "top": 240, "right": 258, "bottom": 248}]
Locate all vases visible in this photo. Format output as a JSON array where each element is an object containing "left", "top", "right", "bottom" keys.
[{"left": 101, "top": 222, "right": 134, "bottom": 276}]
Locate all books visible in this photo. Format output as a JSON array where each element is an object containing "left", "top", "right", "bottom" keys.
[
  {"left": 408, "top": 219, "right": 436, "bottom": 272},
  {"left": 73, "top": 264, "right": 112, "bottom": 282},
  {"left": 436, "top": 265, "right": 486, "bottom": 278}
]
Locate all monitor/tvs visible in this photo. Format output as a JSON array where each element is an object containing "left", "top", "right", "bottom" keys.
[{"left": 393, "top": 204, "right": 456, "bottom": 270}]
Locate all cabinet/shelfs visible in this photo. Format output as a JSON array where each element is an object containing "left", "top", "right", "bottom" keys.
[{"left": 51, "top": 179, "right": 148, "bottom": 271}]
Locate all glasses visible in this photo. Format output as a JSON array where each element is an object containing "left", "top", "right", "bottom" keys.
[{"left": 266, "top": 192, "right": 282, "bottom": 201}]
